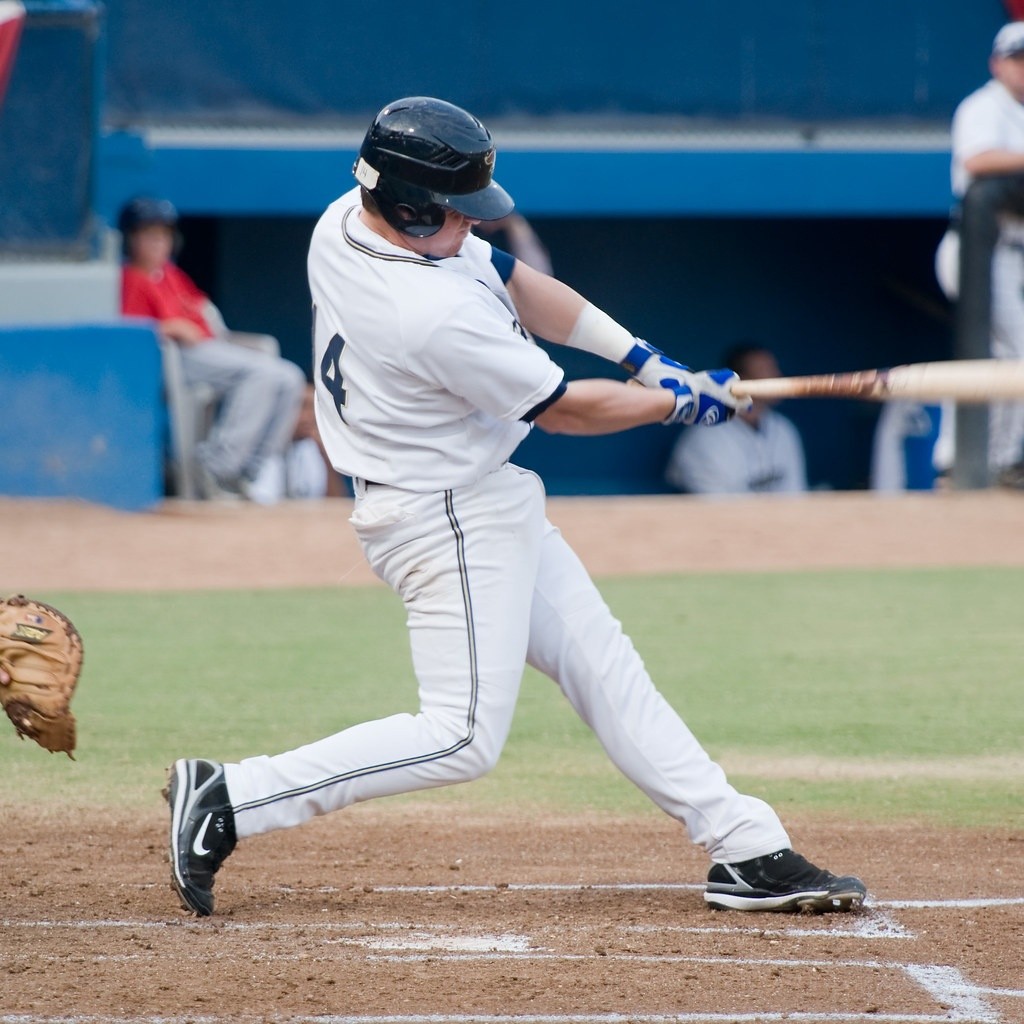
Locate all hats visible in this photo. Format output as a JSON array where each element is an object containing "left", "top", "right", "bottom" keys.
[{"left": 993, "top": 20, "right": 1023, "bottom": 56}]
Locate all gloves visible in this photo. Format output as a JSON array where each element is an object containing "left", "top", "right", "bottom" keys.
[
  {"left": 621, "top": 336, "right": 755, "bottom": 417},
  {"left": 661, "top": 384, "right": 735, "bottom": 428}
]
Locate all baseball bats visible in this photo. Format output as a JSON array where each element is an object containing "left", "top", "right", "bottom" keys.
[{"left": 625, "top": 359, "right": 1023, "bottom": 406}]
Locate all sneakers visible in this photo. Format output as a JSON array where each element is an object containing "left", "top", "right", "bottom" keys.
[
  {"left": 168, "top": 758, "right": 238, "bottom": 918},
  {"left": 703, "top": 847, "right": 866, "bottom": 913}
]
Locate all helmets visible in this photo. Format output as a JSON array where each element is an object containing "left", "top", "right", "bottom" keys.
[
  {"left": 119, "top": 196, "right": 180, "bottom": 232},
  {"left": 353, "top": 96, "right": 516, "bottom": 238}
]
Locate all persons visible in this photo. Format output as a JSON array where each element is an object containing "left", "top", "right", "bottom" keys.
[
  {"left": 119, "top": 195, "right": 341, "bottom": 507},
  {"left": 165, "top": 98, "right": 868, "bottom": 920},
  {"left": 668, "top": 338, "right": 807, "bottom": 492},
  {"left": 937, "top": 23, "right": 1024, "bottom": 487}
]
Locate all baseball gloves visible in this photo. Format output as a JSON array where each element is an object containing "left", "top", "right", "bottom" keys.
[{"left": 1, "top": 594, "right": 83, "bottom": 753}]
